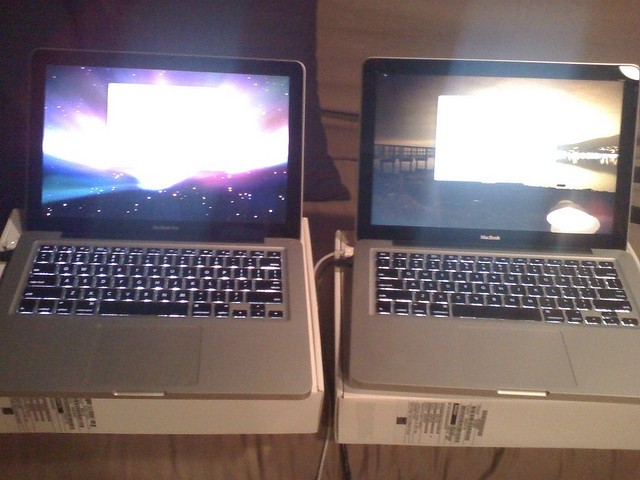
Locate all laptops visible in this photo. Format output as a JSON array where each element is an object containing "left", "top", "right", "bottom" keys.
[
  {"left": 345, "top": 55, "right": 639, "bottom": 404},
  {"left": 0, "top": 44, "right": 312, "bottom": 402}
]
